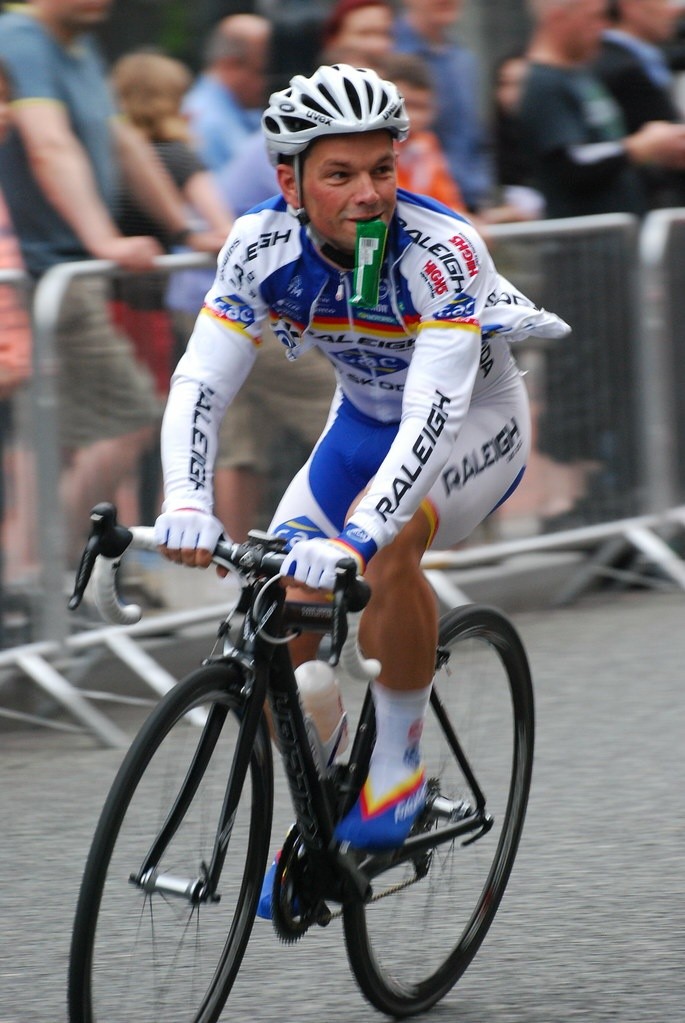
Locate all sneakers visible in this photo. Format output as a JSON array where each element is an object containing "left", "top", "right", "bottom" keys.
[
  {"left": 335, "top": 768, "right": 427, "bottom": 853},
  {"left": 256, "top": 850, "right": 301, "bottom": 919}
]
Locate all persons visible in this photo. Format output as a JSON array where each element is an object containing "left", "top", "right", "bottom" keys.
[
  {"left": 154, "top": 63, "right": 570, "bottom": 918},
  {"left": 0, "top": 0, "right": 684, "bottom": 725}
]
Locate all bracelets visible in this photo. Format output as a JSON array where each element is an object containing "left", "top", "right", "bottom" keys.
[{"left": 173, "top": 218, "right": 213, "bottom": 246}]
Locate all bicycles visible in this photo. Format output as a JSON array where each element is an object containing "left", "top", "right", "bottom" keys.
[{"left": 67, "top": 502, "right": 535, "bottom": 1023}]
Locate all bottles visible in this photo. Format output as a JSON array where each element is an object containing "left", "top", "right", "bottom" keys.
[{"left": 294, "top": 659, "right": 351, "bottom": 758}]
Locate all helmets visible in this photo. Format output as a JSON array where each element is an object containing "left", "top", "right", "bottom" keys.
[{"left": 261, "top": 63, "right": 411, "bottom": 172}]
